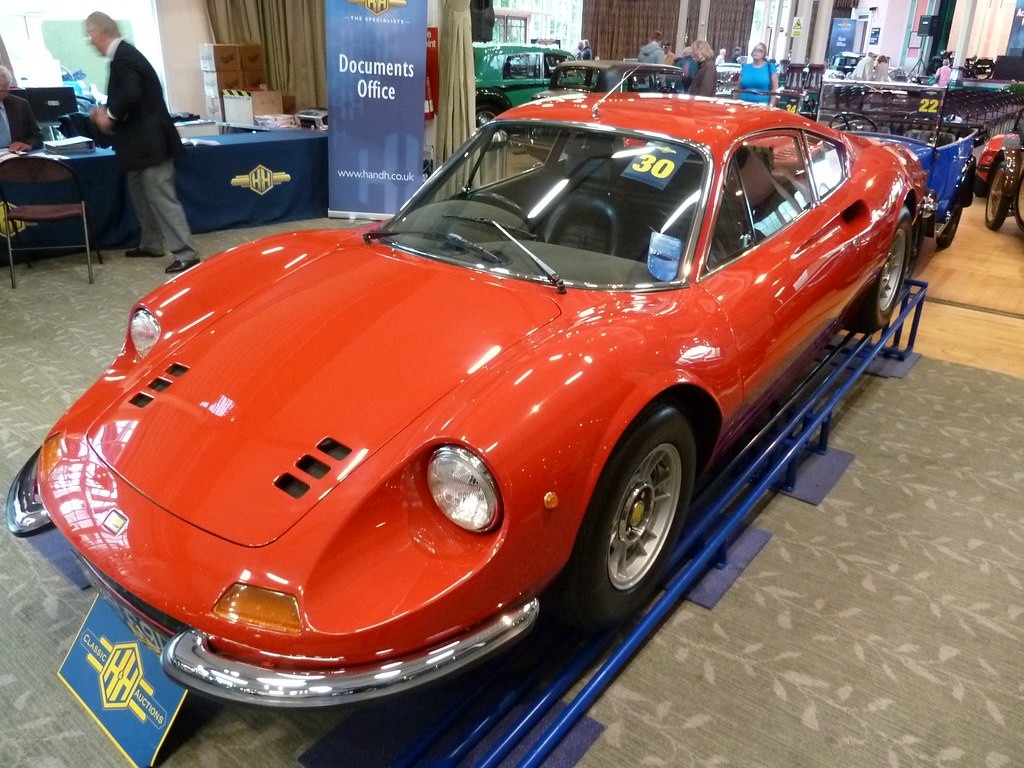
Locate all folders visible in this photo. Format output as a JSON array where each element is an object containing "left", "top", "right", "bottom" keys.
[{"left": 43, "top": 136, "right": 97, "bottom": 155}]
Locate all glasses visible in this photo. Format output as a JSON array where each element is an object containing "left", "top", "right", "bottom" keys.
[{"left": 754, "top": 49, "right": 764, "bottom": 53}]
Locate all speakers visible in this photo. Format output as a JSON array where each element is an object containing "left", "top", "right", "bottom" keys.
[{"left": 917, "top": 16, "right": 940, "bottom": 37}]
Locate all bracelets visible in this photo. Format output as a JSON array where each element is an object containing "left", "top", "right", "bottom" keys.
[{"left": 103, "top": 112, "right": 115, "bottom": 122}]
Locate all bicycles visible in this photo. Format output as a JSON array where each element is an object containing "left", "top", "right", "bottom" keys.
[{"left": 926, "top": 51, "right": 994, "bottom": 80}]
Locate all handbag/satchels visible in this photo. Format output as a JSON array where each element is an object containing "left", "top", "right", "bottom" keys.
[{"left": 928, "top": 75, "right": 938, "bottom": 86}]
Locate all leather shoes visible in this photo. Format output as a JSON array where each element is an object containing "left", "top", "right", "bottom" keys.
[
  {"left": 125, "top": 248, "right": 166, "bottom": 258},
  {"left": 167, "top": 258, "right": 201, "bottom": 274}
]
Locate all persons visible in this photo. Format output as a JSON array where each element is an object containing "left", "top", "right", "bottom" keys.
[
  {"left": 935, "top": 58, "right": 952, "bottom": 88},
  {"left": 87, "top": 11, "right": 201, "bottom": 273},
  {"left": 852, "top": 51, "right": 875, "bottom": 81},
  {"left": 874, "top": 56, "right": 889, "bottom": 81},
  {"left": 0, "top": 66, "right": 43, "bottom": 152},
  {"left": 639, "top": 31, "right": 741, "bottom": 97},
  {"left": 733, "top": 42, "right": 778, "bottom": 109},
  {"left": 576, "top": 41, "right": 592, "bottom": 60}
]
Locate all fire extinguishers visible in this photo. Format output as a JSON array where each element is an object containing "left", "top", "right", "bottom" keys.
[{"left": 424, "top": 75, "right": 435, "bottom": 122}]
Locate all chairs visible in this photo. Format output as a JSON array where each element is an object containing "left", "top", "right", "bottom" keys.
[
  {"left": 847, "top": 115, "right": 973, "bottom": 147},
  {"left": 541, "top": 154, "right": 631, "bottom": 257},
  {"left": 0, "top": 155, "right": 104, "bottom": 290},
  {"left": 618, "top": 162, "right": 731, "bottom": 265}
]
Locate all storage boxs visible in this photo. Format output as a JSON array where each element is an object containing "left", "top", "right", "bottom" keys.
[
  {"left": 223, "top": 91, "right": 283, "bottom": 125},
  {"left": 206, "top": 97, "right": 225, "bottom": 122},
  {"left": 199, "top": 44, "right": 242, "bottom": 72},
  {"left": 245, "top": 68, "right": 267, "bottom": 92},
  {"left": 282, "top": 95, "right": 296, "bottom": 114},
  {"left": 204, "top": 71, "right": 243, "bottom": 96},
  {"left": 241, "top": 45, "right": 265, "bottom": 70}
]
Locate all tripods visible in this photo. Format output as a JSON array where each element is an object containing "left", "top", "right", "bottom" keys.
[{"left": 910, "top": 36, "right": 926, "bottom": 74}]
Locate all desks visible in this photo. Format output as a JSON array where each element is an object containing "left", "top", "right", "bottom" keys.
[{"left": 0, "top": 129, "right": 330, "bottom": 267}]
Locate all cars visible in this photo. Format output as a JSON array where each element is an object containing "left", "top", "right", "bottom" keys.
[
  {"left": 532, "top": 60, "right": 685, "bottom": 102},
  {"left": 985, "top": 146, "right": 1024, "bottom": 231},
  {"left": 829, "top": 53, "right": 867, "bottom": 76},
  {"left": 472, "top": 42, "right": 598, "bottom": 130},
  {"left": 828, "top": 111, "right": 978, "bottom": 246},
  {"left": 974, "top": 107, "right": 1024, "bottom": 198},
  {"left": 6, "top": 93, "right": 929, "bottom": 711}
]
